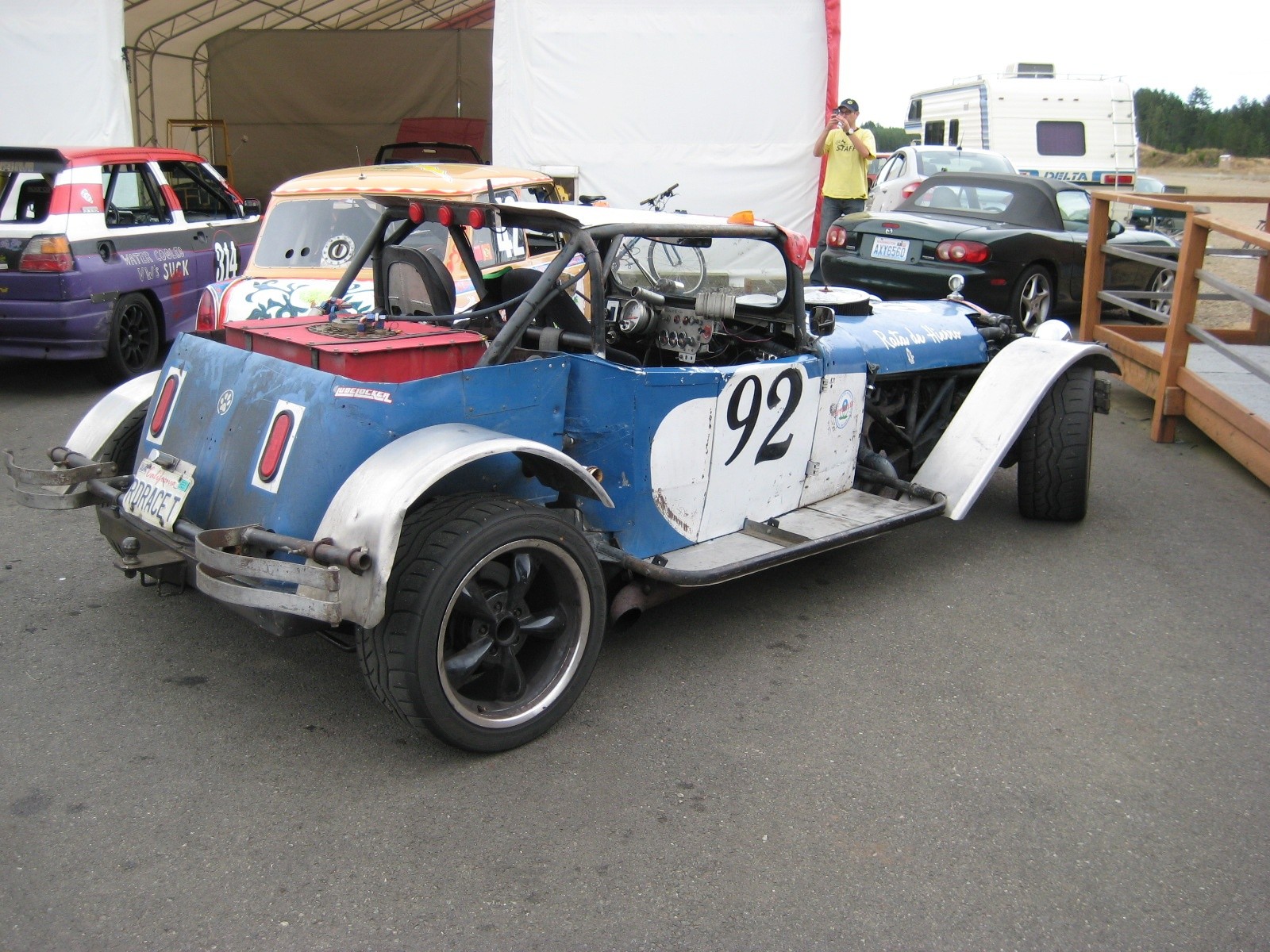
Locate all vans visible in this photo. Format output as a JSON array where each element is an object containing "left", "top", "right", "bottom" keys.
[{"left": 904, "top": 60, "right": 1139, "bottom": 227}]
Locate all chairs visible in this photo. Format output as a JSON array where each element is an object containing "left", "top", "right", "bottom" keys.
[
  {"left": 381, "top": 244, "right": 456, "bottom": 325},
  {"left": 930, "top": 187, "right": 961, "bottom": 208},
  {"left": 498, "top": 267, "right": 640, "bottom": 366},
  {"left": 16, "top": 178, "right": 52, "bottom": 223}
]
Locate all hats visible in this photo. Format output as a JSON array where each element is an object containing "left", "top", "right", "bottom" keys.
[{"left": 838, "top": 98, "right": 859, "bottom": 112}]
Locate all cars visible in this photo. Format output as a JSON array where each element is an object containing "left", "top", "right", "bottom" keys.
[
  {"left": 194, "top": 164, "right": 590, "bottom": 333},
  {"left": 3, "top": 188, "right": 1124, "bottom": 754},
  {"left": 0, "top": 144, "right": 266, "bottom": 384},
  {"left": 866, "top": 145, "right": 1020, "bottom": 215},
  {"left": 820, "top": 172, "right": 1181, "bottom": 337}
]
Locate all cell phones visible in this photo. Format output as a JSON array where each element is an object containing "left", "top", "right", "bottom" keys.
[{"left": 832, "top": 109, "right": 838, "bottom": 118}]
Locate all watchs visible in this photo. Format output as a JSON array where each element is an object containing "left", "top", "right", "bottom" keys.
[{"left": 846, "top": 128, "right": 854, "bottom": 135}]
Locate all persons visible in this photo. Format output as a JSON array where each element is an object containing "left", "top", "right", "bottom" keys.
[{"left": 809, "top": 98, "right": 877, "bottom": 286}]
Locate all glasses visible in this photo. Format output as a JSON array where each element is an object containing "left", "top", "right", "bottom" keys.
[{"left": 839, "top": 110, "right": 854, "bottom": 115}]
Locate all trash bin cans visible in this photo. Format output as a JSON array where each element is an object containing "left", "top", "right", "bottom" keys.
[{"left": 1219, "top": 155, "right": 1231, "bottom": 171}]
